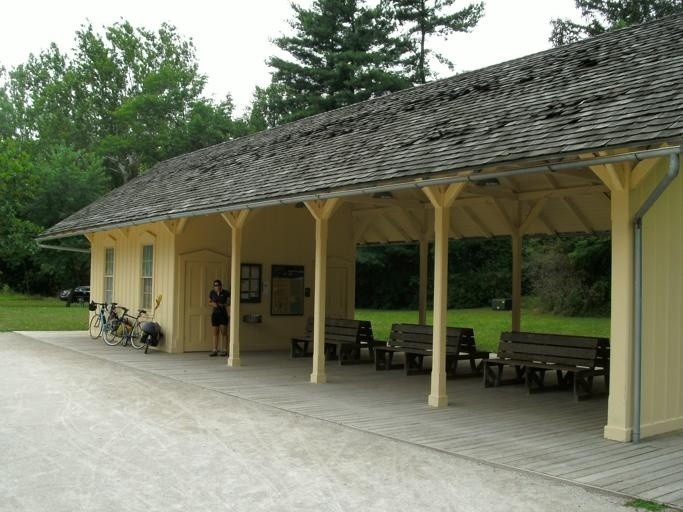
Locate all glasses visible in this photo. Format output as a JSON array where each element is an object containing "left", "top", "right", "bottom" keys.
[{"left": 214, "top": 284, "right": 221, "bottom": 287}]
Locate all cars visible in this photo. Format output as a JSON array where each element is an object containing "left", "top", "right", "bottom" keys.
[{"left": 58, "top": 285, "right": 90, "bottom": 303}]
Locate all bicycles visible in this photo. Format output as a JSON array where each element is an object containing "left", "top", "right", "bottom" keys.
[{"left": 87, "top": 301, "right": 147, "bottom": 349}]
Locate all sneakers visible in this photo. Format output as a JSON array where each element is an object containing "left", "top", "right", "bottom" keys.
[
  {"left": 217, "top": 351, "right": 227, "bottom": 356},
  {"left": 208, "top": 351, "right": 217, "bottom": 356}
]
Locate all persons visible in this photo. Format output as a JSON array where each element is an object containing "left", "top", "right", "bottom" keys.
[{"left": 205, "top": 279, "right": 230, "bottom": 357}]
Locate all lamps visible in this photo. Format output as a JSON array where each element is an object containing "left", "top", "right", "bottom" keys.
[
  {"left": 475, "top": 177, "right": 500, "bottom": 188},
  {"left": 372, "top": 191, "right": 394, "bottom": 200},
  {"left": 293, "top": 202, "right": 305, "bottom": 208}
]
